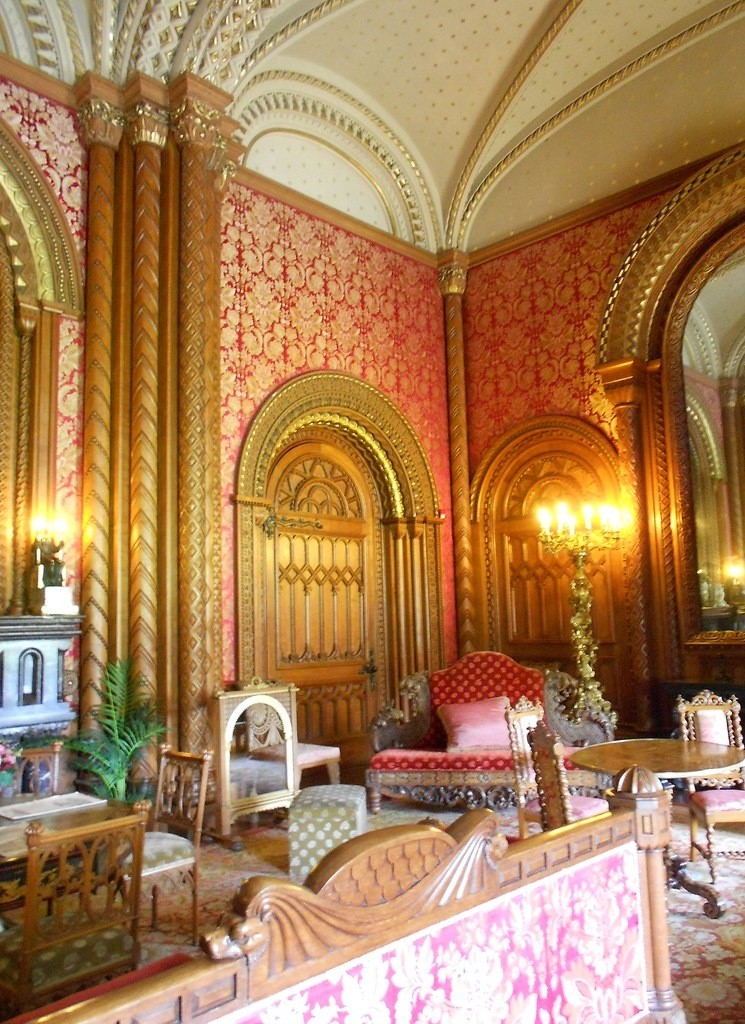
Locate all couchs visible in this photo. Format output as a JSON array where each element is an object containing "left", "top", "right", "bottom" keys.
[{"left": 364, "top": 650, "right": 620, "bottom": 816}]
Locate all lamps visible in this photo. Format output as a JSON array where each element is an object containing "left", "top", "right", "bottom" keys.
[{"left": 536, "top": 499, "right": 637, "bottom": 704}]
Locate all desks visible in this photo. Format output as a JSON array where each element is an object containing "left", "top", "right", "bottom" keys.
[
  {"left": 0, "top": 793, "right": 138, "bottom": 924},
  {"left": 683, "top": 630, "right": 745, "bottom": 700},
  {"left": 567, "top": 737, "right": 745, "bottom": 919}
]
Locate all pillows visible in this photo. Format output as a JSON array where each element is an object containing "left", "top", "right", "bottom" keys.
[{"left": 435, "top": 695, "right": 513, "bottom": 754}]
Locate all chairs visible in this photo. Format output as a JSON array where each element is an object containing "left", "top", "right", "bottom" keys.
[
  {"left": 13, "top": 742, "right": 63, "bottom": 799},
  {"left": 243, "top": 679, "right": 342, "bottom": 791},
  {"left": 674, "top": 688, "right": 745, "bottom": 883},
  {"left": 505, "top": 695, "right": 609, "bottom": 841},
  {"left": 0, "top": 798, "right": 151, "bottom": 1021},
  {"left": 124, "top": 745, "right": 211, "bottom": 946}
]
